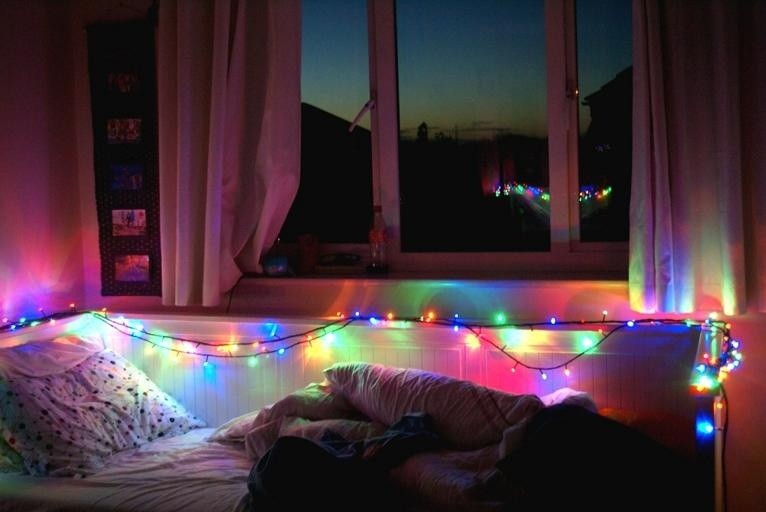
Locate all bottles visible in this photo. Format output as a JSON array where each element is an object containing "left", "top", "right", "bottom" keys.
[{"left": 368, "top": 205, "right": 390, "bottom": 275}]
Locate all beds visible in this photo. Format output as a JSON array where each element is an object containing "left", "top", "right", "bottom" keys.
[{"left": 0, "top": 341, "right": 730, "bottom": 511}]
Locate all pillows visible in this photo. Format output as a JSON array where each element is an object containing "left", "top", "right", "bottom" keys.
[{"left": 1, "top": 333, "right": 208, "bottom": 485}]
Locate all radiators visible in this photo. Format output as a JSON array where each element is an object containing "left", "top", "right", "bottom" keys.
[{"left": 0, "top": 309, "right": 727, "bottom": 449}]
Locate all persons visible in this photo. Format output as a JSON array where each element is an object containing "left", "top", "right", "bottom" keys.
[
  {"left": 120, "top": 211, "right": 138, "bottom": 228},
  {"left": 112, "top": 165, "right": 144, "bottom": 190}
]
